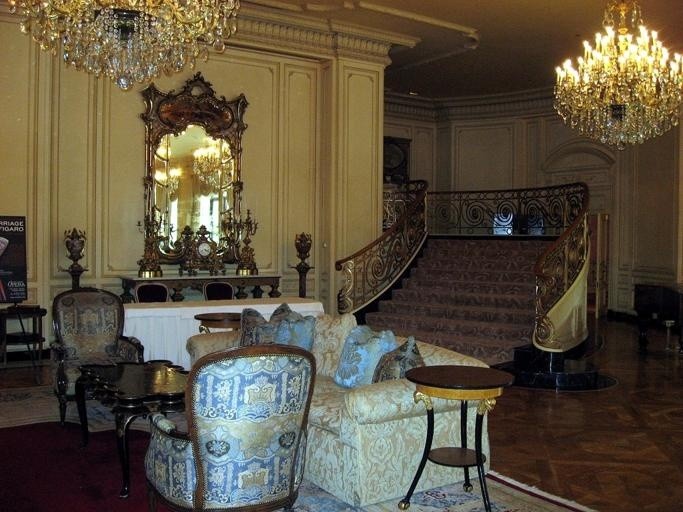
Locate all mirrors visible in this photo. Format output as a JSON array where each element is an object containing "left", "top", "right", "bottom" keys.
[{"left": 138, "top": 72, "right": 249, "bottom": 267}]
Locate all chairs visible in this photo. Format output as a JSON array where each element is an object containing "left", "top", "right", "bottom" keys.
[
  {"left": 1, "top": 303, "right": 48, "bottom": 378},
  {"left": 41, "top": 289, "right": 143, "bottom": 422},
  {"left": 202, "top": 280, "right": 235, "bottom": 301},
  {"left": 137, "top": 280, "right": 169, "bottom": 302},
  {"left": 139, "top": 338, "right": 317, "bottom": 512}
]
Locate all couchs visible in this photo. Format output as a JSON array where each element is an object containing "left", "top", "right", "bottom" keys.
[{"left": 185, "top": 312, "right": 501, "bottom": 506}]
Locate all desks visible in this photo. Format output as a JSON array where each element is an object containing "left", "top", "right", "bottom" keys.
[
  {"left": 91, "top": 289, "right": 328, "bottom": 387},
  {"left": 66, "top": 360, "right": 194, "bottom": 503},
  {"left": 116, "top": 270, "right": 284, "bottom": 305},
  {"left": 399, "top": 360, "right": 518, "bottom": 512}
]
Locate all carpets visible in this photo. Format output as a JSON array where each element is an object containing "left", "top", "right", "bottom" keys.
[{"left": 1, "top": 372, "right": 589, "bottom": 511}]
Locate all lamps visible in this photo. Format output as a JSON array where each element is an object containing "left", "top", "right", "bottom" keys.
[
  {"left": 548, "top": 0, "right": 682, "bottom": 150},
  {"left": 0, "top": 0, "right": 249, "bottom": 97}
]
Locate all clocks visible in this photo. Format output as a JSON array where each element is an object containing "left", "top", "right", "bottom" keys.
[{"left": 178, "top": 222, "right": 225, "bottom": 277}]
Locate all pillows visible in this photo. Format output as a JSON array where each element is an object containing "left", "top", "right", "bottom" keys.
[{"left": 234, "top": 308, "right": 425, "bottom": 387}]
